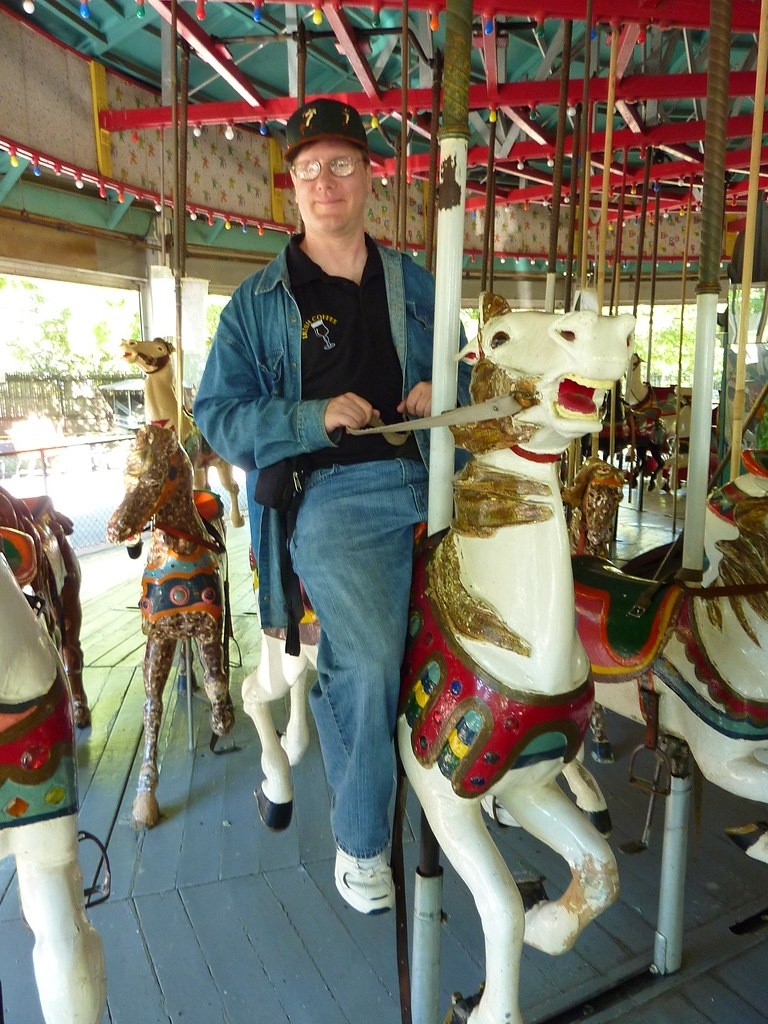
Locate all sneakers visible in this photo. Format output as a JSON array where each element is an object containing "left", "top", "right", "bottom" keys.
[{"left": 333, "top": 840, "right": 398, "bottom": 916}]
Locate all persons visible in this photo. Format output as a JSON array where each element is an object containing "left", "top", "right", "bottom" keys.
[{"left": 192, "top": 98, "right": 519, "bottom": 917}]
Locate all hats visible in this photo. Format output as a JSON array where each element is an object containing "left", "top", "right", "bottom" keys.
[{"left": 282, "top": 98, "right": 369, "bottom": 166}]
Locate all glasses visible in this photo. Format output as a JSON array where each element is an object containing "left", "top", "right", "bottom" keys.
[{"left": 286, "top": 156, "right": 364, "bottom": 181}]
